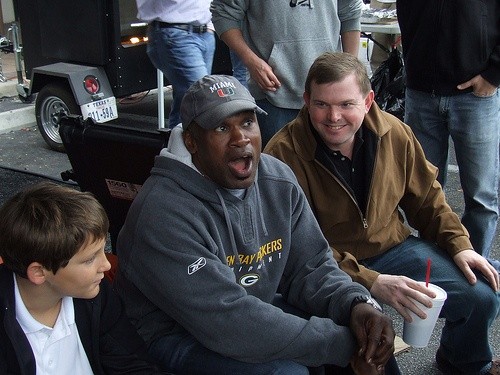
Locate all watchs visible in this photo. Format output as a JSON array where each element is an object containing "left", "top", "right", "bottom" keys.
[{"left": 350, "top": 294, "right": 383, "bottom": 312}]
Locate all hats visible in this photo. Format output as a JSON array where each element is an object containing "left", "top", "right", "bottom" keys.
[{"left": 181, "top": 74, "right": 268, "bottom": 132}]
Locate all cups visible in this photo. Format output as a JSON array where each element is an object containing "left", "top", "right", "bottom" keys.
[{"left": 402, "top": 282, "right": 447, "bottom": 348}]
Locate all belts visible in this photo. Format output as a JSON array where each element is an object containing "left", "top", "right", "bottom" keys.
[{"left": 150, "top": 20, "right": 207, "bottom": 34}]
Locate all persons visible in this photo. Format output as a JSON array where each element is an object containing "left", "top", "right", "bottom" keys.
[
  {"left": 209, "top": 0, "right": 363, "bottom": 153},
  {"left": 396, "top": 0, "right": 500, "bottom": 279},
  {"left": 0, "top": 181, "right": 164, "bottom": 375},
  {"left": 135, "top": 0, "right": 217, "bottom": 129},
  {"left": 370, "top": 0, "right": 405, "bottom": 75},
  {"left": 262, "top": 53, "right": 500, "bottom": 375},
  {"left": 110, "top": 74, "right": 402, "bottom": 375}
]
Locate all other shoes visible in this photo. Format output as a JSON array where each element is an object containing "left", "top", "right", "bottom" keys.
[{"left": 475, "top": 360, "right": 500, "bottom": 375}]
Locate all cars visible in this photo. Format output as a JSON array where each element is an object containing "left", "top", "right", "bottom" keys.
[{"left": 8, "top": 0, "right": 172, "bottom": 152}]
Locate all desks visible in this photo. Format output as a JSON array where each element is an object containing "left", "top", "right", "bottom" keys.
[{"left": 360, "top": 21, "right": 401, "bottom": 56}]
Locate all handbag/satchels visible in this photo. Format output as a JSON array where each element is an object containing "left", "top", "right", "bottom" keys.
[{"left": 360, "top": 31, "right": 406, "bottom": 122}]
